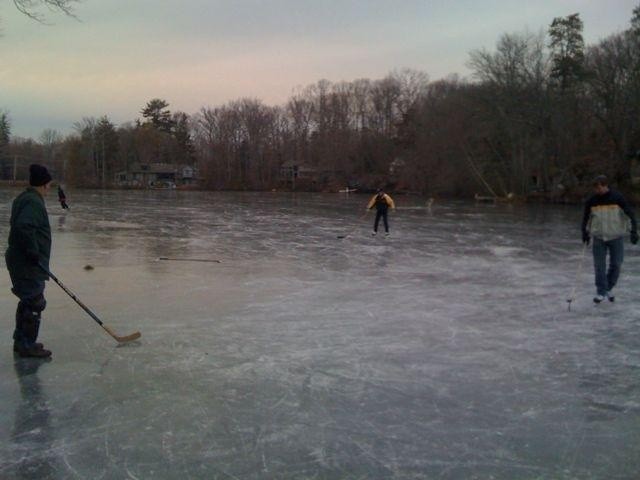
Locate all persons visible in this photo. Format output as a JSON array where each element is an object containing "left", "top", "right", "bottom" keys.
[
  {"left": 4, "top": 161, "right": 55, "bottom": 359},
  {"left": 579, "top": 173, "right": 640, "bottom": 304},
  {"left": 365, "top": 187, "right": 396, "bottom": 237},
  {"left": 9, "top": 360, "right": 59, "bottom": 479},
  {"left": 56, "top": 184, "right": 69, "bottom": 210}
]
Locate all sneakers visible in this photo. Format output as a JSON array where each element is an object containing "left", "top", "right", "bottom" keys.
[{"left": 591, "top": 291, "right": 615, "bottom": 303}]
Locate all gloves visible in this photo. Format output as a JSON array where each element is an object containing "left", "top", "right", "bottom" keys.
[{"left": 26, "top": 250, "right": 44, "bottom": 267}]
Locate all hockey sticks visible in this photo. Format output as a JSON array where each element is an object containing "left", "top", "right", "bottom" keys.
[
  {"left": 566, "top": 242, "right": 586, "bottom": 303},
  {"left": 36, "top": 261, "right": 142, "bottom": 341},
  {"left": 336, "top": 212, "right": 370, "bottom": 239},
  {"left": 159, "top": 257, "right": 223, "bottom": 264}
]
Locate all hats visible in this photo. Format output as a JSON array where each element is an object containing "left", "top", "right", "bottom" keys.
[
  {"left": 375, "top": 187, "right": 383, "bottom": 194},
  {"left": 27, "top": 162, "right": 53, "bottom": 186}
]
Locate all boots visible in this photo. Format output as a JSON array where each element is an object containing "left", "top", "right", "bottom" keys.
[
  {"left": 12, "top": 310, "right": 44, "bottom": 354},
  {"left": 19, "top": 306, "right": 53, "bottom": 358}
]
